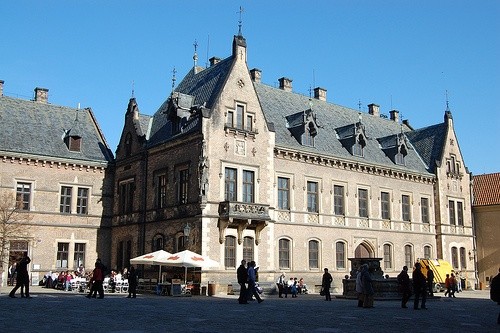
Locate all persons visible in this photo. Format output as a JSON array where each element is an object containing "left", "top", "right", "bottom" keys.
[
  {"left": 322, "top": 268, "right": 333, "bottom": 301},
  {"left": 276, "top": 273, "right": 308, "bottom": 298},
  {"left": 351, "top": 262, "right": 500, "bottom": 324},
  {"left": 237, "top": 259, "right": 265, "bottom": 304},
  {"left": 9, "top": 254, "right": 199, "bottom": 299}
]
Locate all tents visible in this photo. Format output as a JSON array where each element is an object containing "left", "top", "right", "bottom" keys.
[{"left": 130, "top": 250, "right": 220, "bottom": 295}]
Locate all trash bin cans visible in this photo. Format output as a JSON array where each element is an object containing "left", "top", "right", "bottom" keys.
[{"left": 208, "top": 284, "right": 214, "bottom": 295}]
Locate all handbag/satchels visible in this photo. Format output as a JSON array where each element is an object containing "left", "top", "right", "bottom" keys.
[{"left": 320, "top": 291, "right": 325, "bottom": 296}]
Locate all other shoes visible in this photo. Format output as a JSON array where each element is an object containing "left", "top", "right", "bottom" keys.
[
  {"left": 91, "top": 295, "right": 96, "bottom": 298},
  {"left": 26, "top": 296, "right": 32, "bottom": 299},
  {"left": 295, "top": 295, "right": 298, "bottom": 298},
  {"left": 84, "top": 295, "right": 91, "bottom": 299},
  {"left": 414, "top": 307, "right": 421, "bottom": 310},
  {"left": 97, "top": 296, "right": 104, "bottom": 299},
  {"left": 292, "top": 295, "right": 294, "bottom": 298},
  {"left": 8, "top": 294, "right": 17, "bottom": 298},
  {"left": 132, "top": 295, "right": 136, "bottom": 298},
  {"left": 21, "top": 295, "right": 25, "bottom": 298},
  {"left": 279, "top": 295, "right": 283, "bottom": 298},
  {"left": 420, "top": 306, "right": 428, "bottom": 309},
  {"left": 125, "top": 295, "right": 131, "bottom": 298},
  {"left": 238, "top": 298, "right": 265, "bottom": 305}
]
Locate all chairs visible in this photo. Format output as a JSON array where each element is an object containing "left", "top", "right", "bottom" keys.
[{"left": 43, "top": 271, "right": 158, "bottom": 294}]
